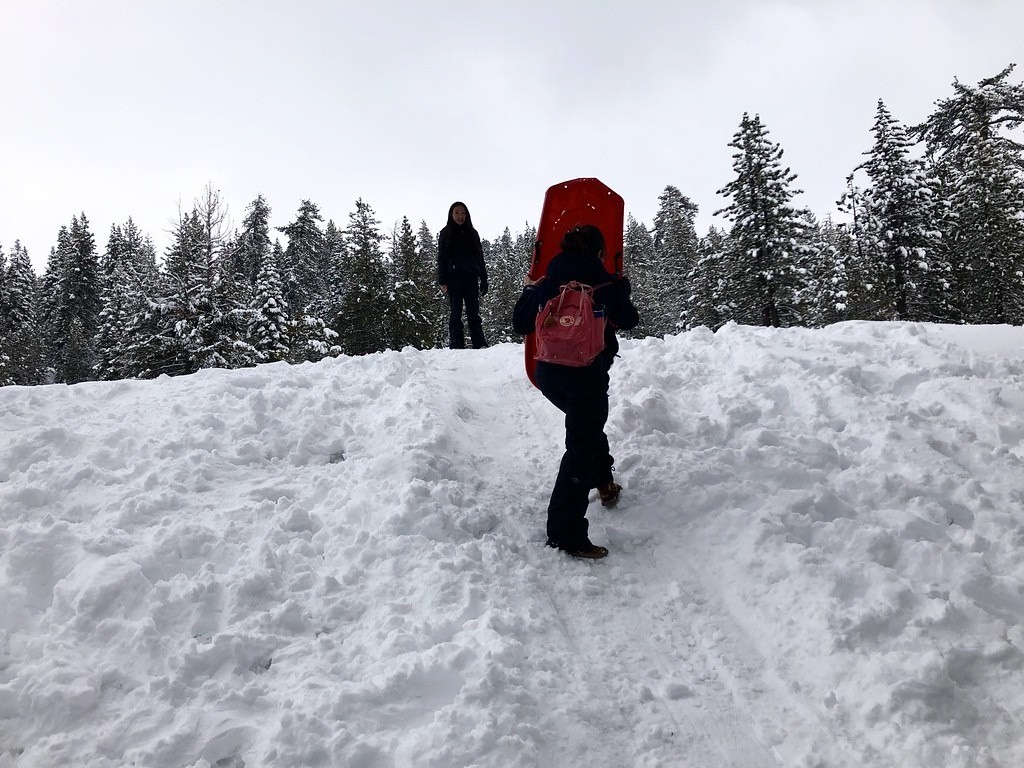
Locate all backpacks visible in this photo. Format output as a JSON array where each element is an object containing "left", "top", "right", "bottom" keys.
[{"left": 532, "top": 281, "right": 610, "bottom": 367}]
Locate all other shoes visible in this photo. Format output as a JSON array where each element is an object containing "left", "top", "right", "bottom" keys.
[
  {"left": 598, "top": 480, "right": 622, "bottom": 506},
  {"left": 546, "top": 536, "right": 609, "bottom": 558}
]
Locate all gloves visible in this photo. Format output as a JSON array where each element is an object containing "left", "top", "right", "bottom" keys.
[{"left": 479, "top": 282, "right": 488, "bottom": 296}]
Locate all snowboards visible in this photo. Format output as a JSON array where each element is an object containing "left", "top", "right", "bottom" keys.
[{"left": 524, "top": 178, "right": 625, "bottom": 393}]
